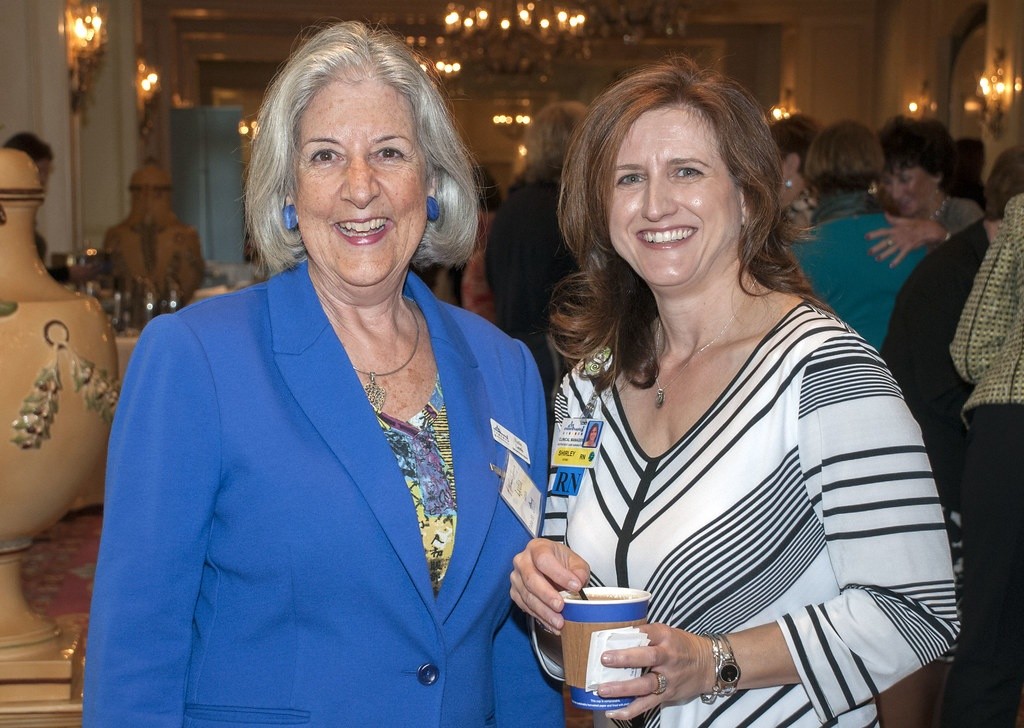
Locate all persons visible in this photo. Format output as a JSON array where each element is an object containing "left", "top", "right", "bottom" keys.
[
  {"left": 80, "top": 20, "right": 568, "bottom": 728},
  {"left": 867, "top": 114, "right": 986, "bottom": 268},
  {"left": 486, "top": 100, "right": 595, "bottom": 432},
  {"left": 882, "top": 145, "right": 1024, "bottom": 517},
  {"left": 411, "top": 153, "right": 538, "bottom": 332},
  {"left": 508, "top": 61, "right": 962, "bottom": 728},
  {"left": 765, "top": 111, "right": 985, "bottom": 246},
  {"left": 941, "top": 194, "right": 1024, "bottom": 728},
  {"left": 2, "top": 132, "right": 100, "bottom": 281},
  {"left": 584, "top": 423, "right": 599, "bottom": 446},
  {"left": 787, "top": 120, "right": 930, "bottom": 356}
]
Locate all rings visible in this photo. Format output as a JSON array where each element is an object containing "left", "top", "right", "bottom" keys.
[
  {"left": 648, "top": 671, "right": 666, "bottom": 694},
  {"left": 888, "top": 238, "right": 893, "bottom": 246}
]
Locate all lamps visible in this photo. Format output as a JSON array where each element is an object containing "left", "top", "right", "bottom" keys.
[{"left": 65, "top": 6, "right": 108, "bottom": 109}]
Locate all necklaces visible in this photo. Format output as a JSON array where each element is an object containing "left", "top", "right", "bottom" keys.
[
  {"left": 654, "top": 279, "right": 757, "bottom": 406},
  {"left": 352, "top": 294, "right": 420, "bottom": 411},
  {"left": 928, "top": 195, "right": 951, "bottom": 221}
]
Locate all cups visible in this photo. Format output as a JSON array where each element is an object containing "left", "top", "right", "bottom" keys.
[{"left": 527, "top": 586, "right": 651, "bottom": 711}]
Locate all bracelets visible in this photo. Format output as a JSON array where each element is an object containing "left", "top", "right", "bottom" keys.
[{"left": 945, "top": 230, "right": 950, "bottom": 240}]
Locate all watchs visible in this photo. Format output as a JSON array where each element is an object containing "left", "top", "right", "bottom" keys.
[{"left": 701, "top": 630, "right": 740, "bottom": 706}]
